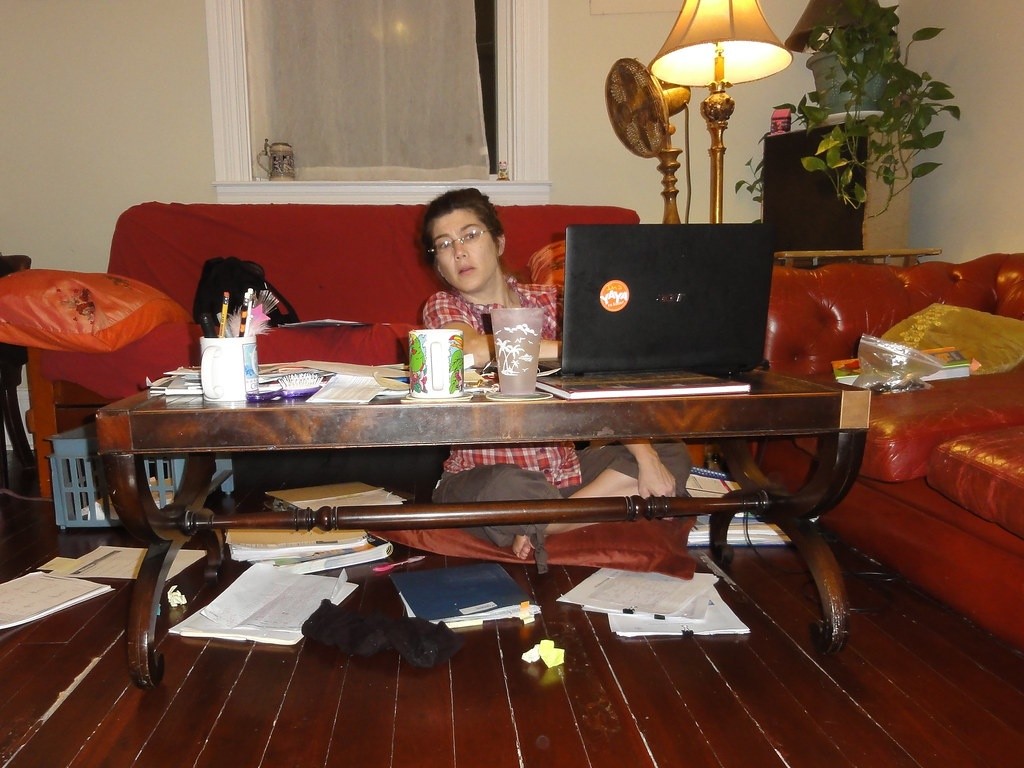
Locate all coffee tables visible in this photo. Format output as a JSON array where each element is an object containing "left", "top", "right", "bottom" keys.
[{"left": 84, "top": 368, "right": 880, "bottom": 693}]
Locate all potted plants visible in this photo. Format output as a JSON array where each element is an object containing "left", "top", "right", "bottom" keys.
[{"left": 733, "top": 5, "right": 964, "bottom": 238}]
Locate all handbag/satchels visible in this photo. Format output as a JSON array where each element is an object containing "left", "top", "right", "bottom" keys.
[{"left": 191, "top": 256, "right": 301, "bottom": 325}]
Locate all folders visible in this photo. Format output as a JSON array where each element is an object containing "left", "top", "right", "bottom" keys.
[{"left": 389, "top": 562, "right": 541, "bottom": 627}]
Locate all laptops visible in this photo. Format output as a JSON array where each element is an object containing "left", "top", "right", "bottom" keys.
[{"left": 535, "top": 222, "right": 777, "bottom": 378}]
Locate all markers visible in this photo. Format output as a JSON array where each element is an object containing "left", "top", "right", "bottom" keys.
[{"left": 200, "top": 314, "right": 219, "bottom": 338}]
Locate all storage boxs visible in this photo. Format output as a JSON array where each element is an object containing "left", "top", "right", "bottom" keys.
[{"left": 41, "top": 422, "right": 235, "bottom": 533}]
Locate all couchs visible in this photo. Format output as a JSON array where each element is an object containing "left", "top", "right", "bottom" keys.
[
  {"left": 22, "top": 201, "right": 680, "bottom": 503},
  {"left": 765, "top": 249, "right": 1024, "bottom": 651}
]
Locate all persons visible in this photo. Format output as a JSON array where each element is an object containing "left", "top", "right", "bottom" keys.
[{"left": 422, "top": 188, "right": 692, "bottom": 559}]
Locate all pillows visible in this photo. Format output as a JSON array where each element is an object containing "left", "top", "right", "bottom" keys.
[
  {"left": 526, "top": 238, "right": 566, "bottom": 287},
  {"left": 0, "top": 268, "right": 195, "bottom": 355}
]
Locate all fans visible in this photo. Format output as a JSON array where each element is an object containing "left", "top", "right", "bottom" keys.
[{"left": 604, "top": 57, "right": 692, "bottom": 222}]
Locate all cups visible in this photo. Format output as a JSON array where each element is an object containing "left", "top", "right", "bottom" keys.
[
  {"left": 199, "top": 337, "right": 259, "bottom": 404},
  {"left": 257, "top": 139, "right": 297, "bottom": 181},
  {"left": 409, "top": 329, "right": 464, "bottom": 399},
  {"left": 490, "top": 308, "right": 543, "bottom": 396}
]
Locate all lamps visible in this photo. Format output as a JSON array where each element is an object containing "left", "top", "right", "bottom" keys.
[
  {"left": 785, "top": 0, "right": 898, "bottom": 54},
  {"left": 647, "top": 0, "right": 794, "bottom": 225}
]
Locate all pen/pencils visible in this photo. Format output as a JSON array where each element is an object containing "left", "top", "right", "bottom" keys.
[
  {"left": 245, "top": 288, "right": 253, "bottom": 337},
  {"left": 217, "top": 313, "right": 233, "bottom": 338},
  {"left": 373, "top": 555, "right": 425, "bottom": 572},
  {"left": 239, "top": 293, "right": 250, "bottom": 337},
  {"left": 219, "top": 292, "right": 230, "bottom": 337}
]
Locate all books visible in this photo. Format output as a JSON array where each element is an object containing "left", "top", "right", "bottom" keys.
[
  {"left": 536, "top": 371, "right": 751, "bottom": 401},
  {"left": 685, "top": 467, "right": 793, "bottom": 546},
  {"left": 389, "top": 563, "right": 542, "bottom": 628},
  {"left": 831, "top": 347, "right": 971, "bottom": 386},
  {"left": 149, "top": 361, "right": 499, "bottom": 395},
  {"left": 0, "top": 571, "right": 116, "bottom": 629},
  {"left": 224, "top": 482, "right": 407, "bottom": 574}
]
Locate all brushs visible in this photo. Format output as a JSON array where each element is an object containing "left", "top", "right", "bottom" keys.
[{"left": 245, "top": 374, "right": 324, "bottom": 402}]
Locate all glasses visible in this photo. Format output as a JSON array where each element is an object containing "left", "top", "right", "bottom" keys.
[{"left": 427, "top": 228, "right": 493, "bottom": 254}]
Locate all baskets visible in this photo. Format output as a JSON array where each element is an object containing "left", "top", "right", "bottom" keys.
[{"left": 43, "top": 424, "right": 264, "bottom": 528}]
